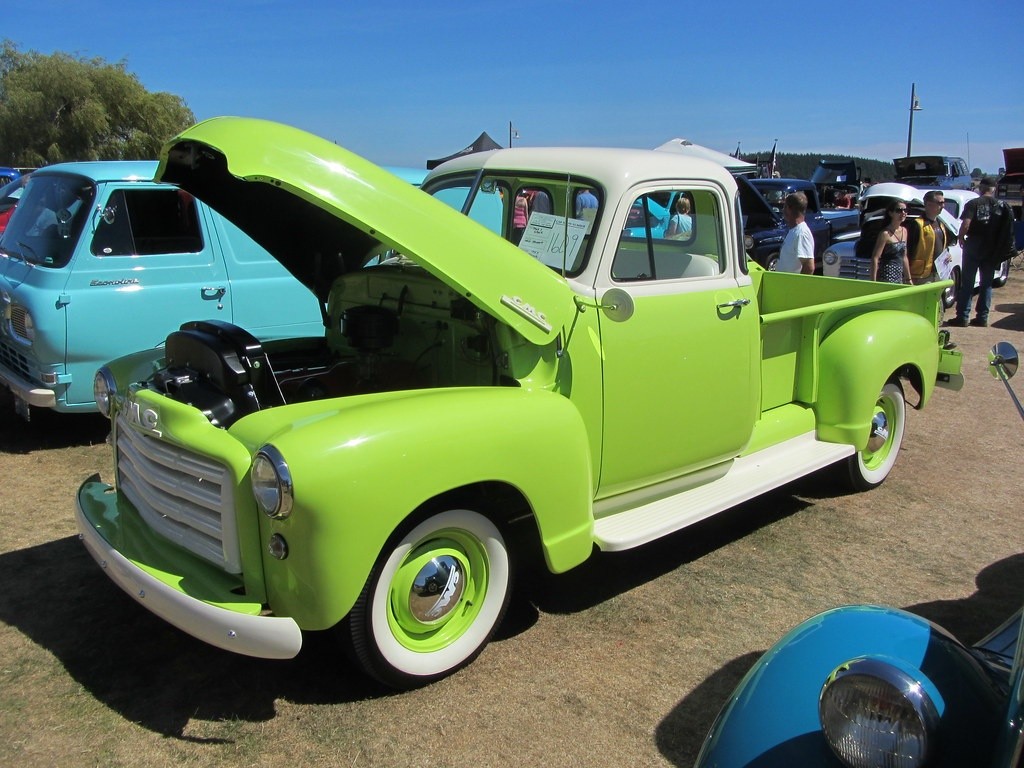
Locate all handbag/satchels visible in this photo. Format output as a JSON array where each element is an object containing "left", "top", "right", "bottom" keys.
[{"left": 664, "top": 230, "right": 672, "bottom": 238}]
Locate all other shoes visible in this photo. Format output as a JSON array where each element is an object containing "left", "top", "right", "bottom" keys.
[
  {"left": 970, "top": 318, "right": 987, "bottom": 327},
  {"left": 947, "top": 317, "right": 969, "bottom": 328}
]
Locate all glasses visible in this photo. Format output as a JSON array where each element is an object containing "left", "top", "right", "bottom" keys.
[
  {"left": 928, "top": 199, "right": 944, "bottom": 205},
  {"left": 892, "top": 208, "right": 910, "bottom": 214}
]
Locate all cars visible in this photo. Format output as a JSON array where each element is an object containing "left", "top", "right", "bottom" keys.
[
  {"left": 690, "top": 602, "right": 1024, "bottom": 768},
  {"left": 1, "top": 149, "right": 1023, "bottom": 433}
]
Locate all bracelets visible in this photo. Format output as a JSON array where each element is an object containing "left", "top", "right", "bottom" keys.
[{"left": 905, "top": 278, "right": 912, "bottom": 281}]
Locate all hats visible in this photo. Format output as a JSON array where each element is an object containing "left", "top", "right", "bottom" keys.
[{"left": 979, "top": 177, "right": 998, "bottom": 188}]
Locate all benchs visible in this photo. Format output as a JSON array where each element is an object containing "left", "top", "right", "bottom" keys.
[{"left": 572, "top": 244, "right": 721, "bottom": 281}]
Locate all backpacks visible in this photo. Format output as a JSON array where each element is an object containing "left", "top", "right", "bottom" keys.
[{"left": 986, "top": 198, "right": 1018, "bottom": 265}]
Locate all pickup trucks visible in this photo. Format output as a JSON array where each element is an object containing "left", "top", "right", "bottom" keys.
[{"left": 73, "top": 116, "right": 964, "bottom": 694}]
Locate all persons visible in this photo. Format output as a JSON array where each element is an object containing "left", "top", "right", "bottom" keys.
[
  {"left": 499, "top": 188, "right": 549, "bottom": 247},
  {"left": 669, "top": 198, "right": 692, "bottom": 235},
  {"left": 770, "top": 170, "right": 872, "bottom": 275},
  {"left": 948, "top": 178, "right": 1004, "bottom": 327},
  {"left": 0, "top": 173, "right": 58, "bottom": 240},
  {"left": 870, "top": 191, "right": 945, "bottom": 285},
  {"left": 576, "top": 188, "right": 598, "bottom": 221}
]
